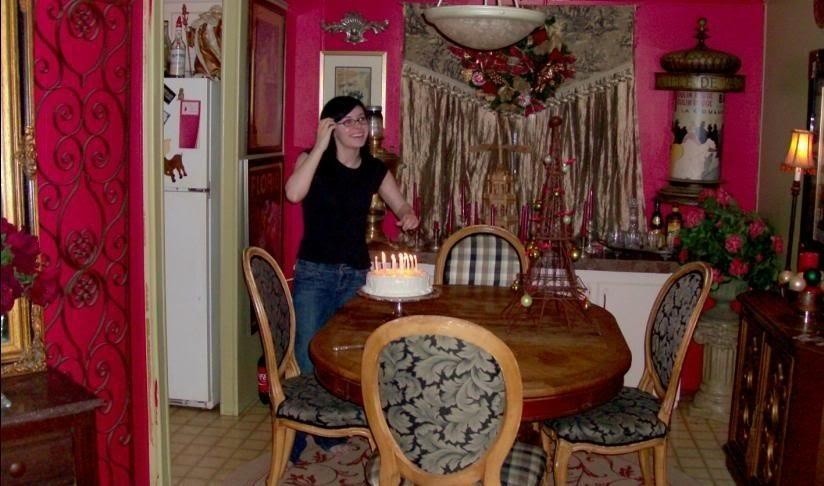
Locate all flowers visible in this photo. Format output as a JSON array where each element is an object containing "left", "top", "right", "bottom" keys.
[
  {"left": 0, "top": 217, "right": 56, "bottom": 313},
  {"left": 444, "top": 26, "right": 576, "bottom": 116}
]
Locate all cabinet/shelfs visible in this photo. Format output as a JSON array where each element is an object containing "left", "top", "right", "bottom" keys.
[
  {"left": 722, "top": 289, "right": 824, "bottom": 486},
  {"left": 0, "top": 373, "right": 106, "bottom": 485}
]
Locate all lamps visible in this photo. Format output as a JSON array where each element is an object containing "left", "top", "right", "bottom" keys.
[
  {"left": 422, "top": 0, "right": 546, "bottom": 52},
  {"left": 773, "top": 128, "right": 817, "bottom": 298}
]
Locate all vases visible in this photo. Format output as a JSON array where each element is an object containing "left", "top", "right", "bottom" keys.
[{"left": 704, "top": 275, "right": 748, "bottom": 324}]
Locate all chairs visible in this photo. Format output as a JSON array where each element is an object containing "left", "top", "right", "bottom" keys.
[
  {"left": 241, "top": 246, "right": 377, "bottom": 486},
  {"left": 361, "top": 315, "right": 548, "bottom": 486},
  {"left": 434, "top": 224, "right": 531, "bottom": 287},
  {"left": 539, "top": 262, "right": 713, "bottom": 486}
]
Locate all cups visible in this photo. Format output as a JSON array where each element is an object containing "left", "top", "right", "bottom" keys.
[
  {"left": 608, "top": 230, "right": 625, "bottom": 249},
  {"left": 642, "top": 233, "right": 664, "bottom": 251},
  {"left": 625, "top": 231, "right": 642, "bottom": 249}
]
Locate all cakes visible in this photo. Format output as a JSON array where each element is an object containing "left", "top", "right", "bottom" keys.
[{"left": 363, "top": 267, "right": 433, "bottom": 297}]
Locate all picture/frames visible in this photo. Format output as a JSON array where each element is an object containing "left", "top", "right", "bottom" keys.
[
  {"left": 245, "top": 0, "right": 287, "bottom": 155},
  {"left": 238, "top": 154, "right": 285, "bottom": 270},
  {"left": 0, "top": 0, "right": 49, "bottom": 379},
  {"left": 319, "top": 49, "right": 387, "bottom": 130}
]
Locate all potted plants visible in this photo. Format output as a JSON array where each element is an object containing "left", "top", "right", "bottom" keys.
[{"left": 671, "top": 188, "right": 784, "bottom": 281}]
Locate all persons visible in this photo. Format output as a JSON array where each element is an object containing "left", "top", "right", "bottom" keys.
[{"left": 285, "top": 96, "right": 420, "bottom": 472}]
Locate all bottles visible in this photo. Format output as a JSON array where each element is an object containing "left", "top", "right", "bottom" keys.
[
  {"left": 171, "top": 16, "right": 186, "bottom": 78},
  {"left": 163, "top": 20, "right": 173, "bottom": 77},
  {"left": 667, "top": 202, "right": 682, "bottom": 247},
  {"left": 650, "top": 200, "right": 663, "bottom": 250}
]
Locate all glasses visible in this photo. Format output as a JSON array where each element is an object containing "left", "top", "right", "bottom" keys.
[{"left": 333, "top": 115, "right": 371, "bottom": 127}]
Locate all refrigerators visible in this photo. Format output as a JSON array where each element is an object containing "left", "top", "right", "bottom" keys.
[{"left": 160, "top": 76, "right": 220, "bottom": 411}]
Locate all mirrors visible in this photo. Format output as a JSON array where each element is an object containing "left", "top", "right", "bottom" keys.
[{"left": 797, "top": 47, "right": 823, "bottom": 270}]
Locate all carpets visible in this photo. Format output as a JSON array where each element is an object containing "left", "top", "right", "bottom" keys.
[{"left": 220, "top": 436, "right": 710, "bottom": 486}]
